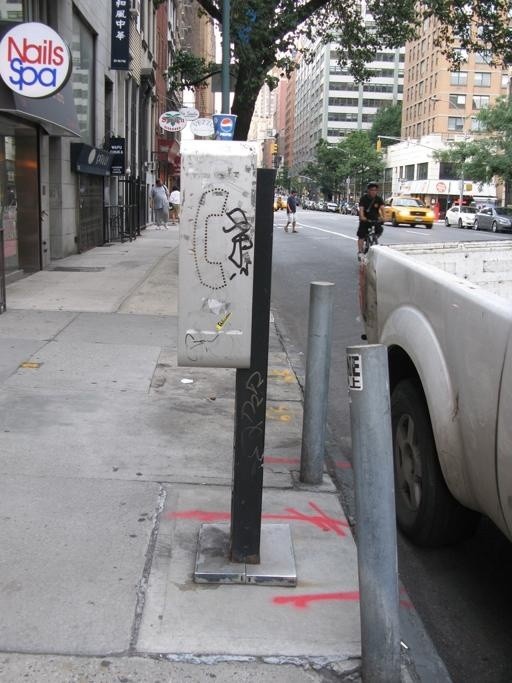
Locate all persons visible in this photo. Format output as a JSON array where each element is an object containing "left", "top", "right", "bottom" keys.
[
  {"left": 284, "top": 189, "right": 300, "bottom": 233},
  {"left": 355, "top": 182, "right": 387, "bottom": 260},
  {"left": 169, "top": 185, "right": 181, "bottom": 224},
  {"left": 150, "top": 178, "right": 170, "bottom": 229}
]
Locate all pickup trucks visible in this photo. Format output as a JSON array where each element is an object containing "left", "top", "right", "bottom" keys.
[{"left": 358, "top": 240, "right": 512, "bottom": 553}]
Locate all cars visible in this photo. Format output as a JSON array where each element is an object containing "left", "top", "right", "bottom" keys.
[
  {"left": 474, "top": 205, "right": 512, "bottom": 232},
  {"left": 445, "top": 206, "right": 479, "bottom": 228},
  {"left": 301, "top": 200, "right": 360, "bottom": 216},
  {"left": 273, "top": 194, "right": 299, "bottom": 212},
  {"left": 380, "top": 193, "right": 435, "bottom": 229}
]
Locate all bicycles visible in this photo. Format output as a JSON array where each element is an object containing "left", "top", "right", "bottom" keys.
[{"left": 362, "top": 218, "right": 383, "bottom": 255}]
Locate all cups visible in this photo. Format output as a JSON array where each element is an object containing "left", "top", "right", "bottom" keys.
[{"left": 212, "top": 114, "right": 237, "bottom": 140}]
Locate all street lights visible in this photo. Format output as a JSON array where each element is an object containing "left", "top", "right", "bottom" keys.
[{"left": 429, "top": 97, "right": 466, "bottom": 206}]
